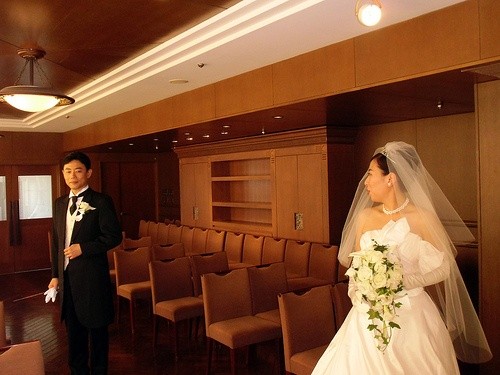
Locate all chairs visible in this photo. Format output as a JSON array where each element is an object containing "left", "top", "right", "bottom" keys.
[{"left": 107, "top": 218, "right": 353, "bottom": 375}]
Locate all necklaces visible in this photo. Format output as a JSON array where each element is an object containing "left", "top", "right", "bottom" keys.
[{"left": 382, "top": 197, "right": 410, "bottom": 215}]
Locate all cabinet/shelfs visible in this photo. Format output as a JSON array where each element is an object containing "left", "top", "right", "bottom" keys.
[{"left": 172, "top": 126, "right": 358, "bottom": 247}]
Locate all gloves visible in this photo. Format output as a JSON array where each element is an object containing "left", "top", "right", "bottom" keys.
[{"left": 44, "top": 286, "right": 59, "bottom": 303}]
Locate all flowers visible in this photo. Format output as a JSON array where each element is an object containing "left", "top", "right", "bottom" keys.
[
  {"left": 344, "top": 239, "right": 404, "bottom": 353},
  {"left": 78, "top": 201, "right": 96, "bottom": 212}
]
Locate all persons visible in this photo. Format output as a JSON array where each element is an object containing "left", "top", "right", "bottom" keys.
[
  {"left": 47, "top": 152, "right": 123, "bottom": 375},
  {"left": 310, "top": 143, "right": 459, "bottom": 375}
]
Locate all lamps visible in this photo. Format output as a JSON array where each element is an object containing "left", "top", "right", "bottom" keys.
[{"left": 0, "top": 48, "right": 75, "bottom": 112}]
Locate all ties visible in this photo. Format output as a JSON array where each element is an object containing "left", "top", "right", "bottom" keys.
[{"left": 70, "top": 196, "right": 78, "bottom": 216}]
